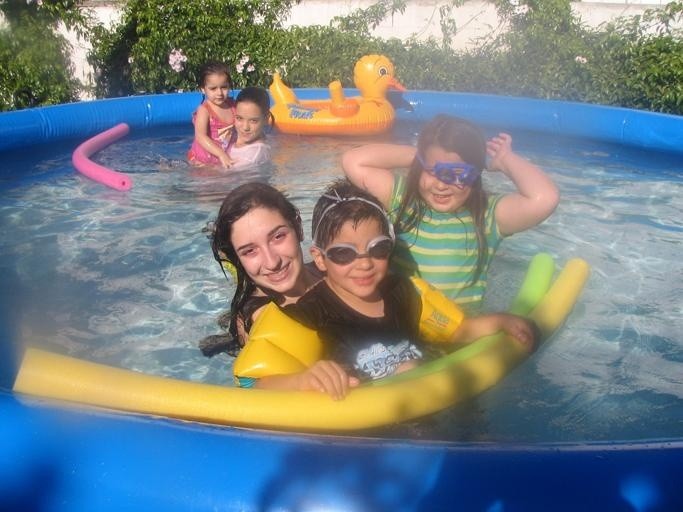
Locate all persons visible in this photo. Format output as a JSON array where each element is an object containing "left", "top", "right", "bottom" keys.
[
  {"left": 187, "top": 62, "right": 238, "bottom": 169},
  {"left": 224, "top": 87, "right": 274, "bottom": 170},
  {"left": 236, "top": 180, "right": 539, "bottom": 401},
  {"left": 340, "top": 116, "right": 560, "bottom": 312},
  {"left": 201, "top": 181, "right": 324, "bottom": 357}
]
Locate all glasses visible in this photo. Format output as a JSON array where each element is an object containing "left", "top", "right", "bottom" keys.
[
  {"left": 416, "top": 151, "right": 482, "bottom": 190},
  {"left": 312, "top": 190, "right": 396, "bottom": 266}
]
